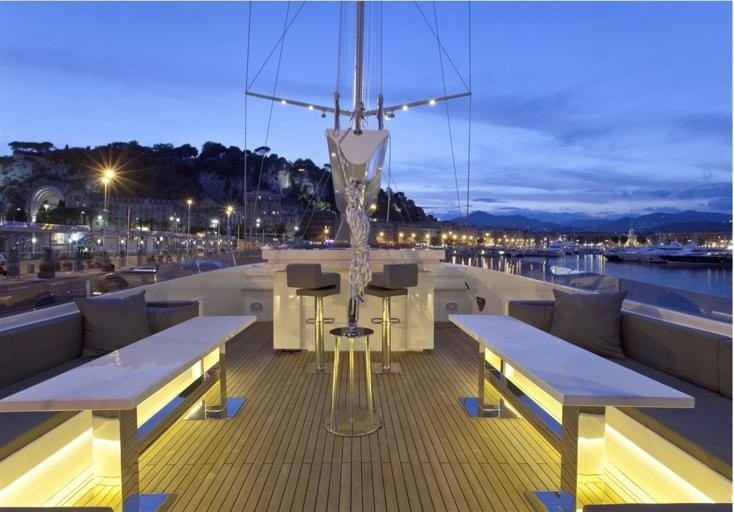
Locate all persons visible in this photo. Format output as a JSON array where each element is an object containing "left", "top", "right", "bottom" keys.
[{"left": 91, "top": 263, "right": 128, "bottom": 297}]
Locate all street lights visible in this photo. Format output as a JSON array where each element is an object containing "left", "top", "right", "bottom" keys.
[
  {"left": 224, "top": 205, "right": 235, "bottom": 237},
  {"left": 187, "top": 198, "right": 193, "bottom": 249},
  {"left": 101, "top": 169, "right": 116, "bottom": 268}
]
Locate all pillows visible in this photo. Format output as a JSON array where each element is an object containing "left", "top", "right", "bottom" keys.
[
  {"left": 73, "top": 289, "right": 152, "bottom": 361},
  {"left": 548, "top": 289, "right": 629, "bottom": 360}
]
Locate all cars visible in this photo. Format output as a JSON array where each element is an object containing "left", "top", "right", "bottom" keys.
[{"left": 548, "top": 244, "right": 564, "bottom": 255}]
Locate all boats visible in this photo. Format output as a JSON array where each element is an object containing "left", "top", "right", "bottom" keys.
[{"left": 603, "top": 244, "right": 732, "bottom": 266}]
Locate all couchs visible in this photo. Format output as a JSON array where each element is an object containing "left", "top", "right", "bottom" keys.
[
  {"left": 506, "top": 299, "right": 732, "bottom": 505},
  {"left": 0, "top": 297, "right": 205, "bottom": 498}
]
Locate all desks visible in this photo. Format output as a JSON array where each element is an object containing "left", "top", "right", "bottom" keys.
[
  {"left": 0, "top": 315, "right": 258, "bottom": 512},
  {"left": 447, "top": 313, "right": 696, "bottom": 511}
]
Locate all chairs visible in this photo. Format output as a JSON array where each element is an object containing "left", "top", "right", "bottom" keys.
[
  {"left": 286, "top": 262, "right": 340, "bottom": 374},
  {"left": 363, "top": 263, "right": 418, "bottom": 375}
]
